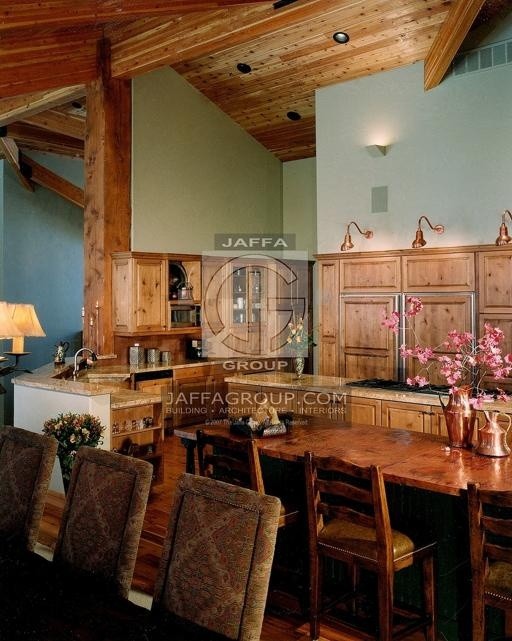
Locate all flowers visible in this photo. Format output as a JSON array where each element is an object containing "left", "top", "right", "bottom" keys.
[
  {"left": 40, "top": 410, "right": 108, "bottom": 493},
  {"left": 375, "top": 293, "right": 512, "bottom": 412},
  {"left": 283, "top": 316, "right": 317, "bottom": 351}
]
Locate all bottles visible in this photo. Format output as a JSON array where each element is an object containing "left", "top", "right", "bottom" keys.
[
  {"left": 55, "top": 354, "right": 62, "bottom": 366},
  {"left": 129, "top": 343, "right": 148, "bottom": 366},
  {"left": 236, "top": 270, "right": 243, "bottom": 292}
]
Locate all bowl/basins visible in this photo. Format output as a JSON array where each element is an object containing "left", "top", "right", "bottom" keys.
[{"left": 172, "top": 310, "right": 194, "bottom": 323}]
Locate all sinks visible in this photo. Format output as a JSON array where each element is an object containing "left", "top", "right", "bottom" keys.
[{"left": 78, "top": 374, "right": 131, "bottom": 383}]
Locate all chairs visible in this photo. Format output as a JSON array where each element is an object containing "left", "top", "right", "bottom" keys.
[{"left": 0, "top": 424, "right": 512, "bottom": 641}]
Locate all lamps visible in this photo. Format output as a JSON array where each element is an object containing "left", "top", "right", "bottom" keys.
[
  {"left": 1, "top": 300, "right": 45, "bottom": 394},
  {"left": 494, "top": 209, "right": 512, "bottom": 247},
  {"left": 341, "top": 221, "right": 374, "bottom": 253},
  {"left": 411, "top": 214, "right": 447, "bottom": 248}
]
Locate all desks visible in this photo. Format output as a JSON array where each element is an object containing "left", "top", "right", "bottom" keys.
[{"left": 172, "top": 410, "right": 512, "bottom": 630}]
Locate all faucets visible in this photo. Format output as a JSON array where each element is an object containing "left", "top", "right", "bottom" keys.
[{"left": 72, "top": 347, "right": 98, "bottom": 376}]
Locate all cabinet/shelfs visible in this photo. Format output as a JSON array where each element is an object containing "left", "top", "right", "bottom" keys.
[
  {"left": 298, "top": 391, "right": 382, "bottom": 430},
  {"left": 229, "top": 258, "right": 267, "bottom": 332},
  {"left": 258, "top": 258, "right": 313, "bottom": 357},
  {"left": 109, "top": 249, "right": 169, "bottom": 337},
  {"left": 164, "top": 252, "right": 228, "bottom": 334},
  {"left": 310, "top": 240, "right": 512, "bottom": 392},
  {"left": 226, "top": 383, "right": 298, "bottom": 419},
  {"left": 172, "top": 360, "right": 237, "bottom": 425},
  {"left": 111, "top": 404, "right": 165, "bottom": 486},
  {"left": 483, "top": 411, "right": 512, "bottom": 450},
  {"left": 382, "top": 401, "right": 483, "bottom": 445},
  {"left": 238, "top": 358, "right": 295, "bottom": 376}
]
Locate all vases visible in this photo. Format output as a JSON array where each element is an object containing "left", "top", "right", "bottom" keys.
[
  {"left": 444, "top": 387, "right": 475, "bottom": 448},
  {"left": 291, "top": 350, "right": 307, "bottom": 381}
]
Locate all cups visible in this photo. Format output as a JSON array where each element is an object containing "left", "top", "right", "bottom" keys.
[
  {"left": 233, "top": 304, "right": 238, "bottom": 310},
  {"left": 147, "top": 347, "right": 160, "bottom": 363},
  {"left": 236, "top": 298, "right": 243, "bottom": 310},
  {"left": 161, "top": 351, "right": 173, "bottom": 363}
]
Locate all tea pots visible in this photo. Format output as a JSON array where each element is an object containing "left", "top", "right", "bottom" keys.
[
  {"left": 477, "top": 410, "right": 511, "bottom": 458},
  {"left": 54, "top": 341, "right": 70, "bottom": 366}
]
